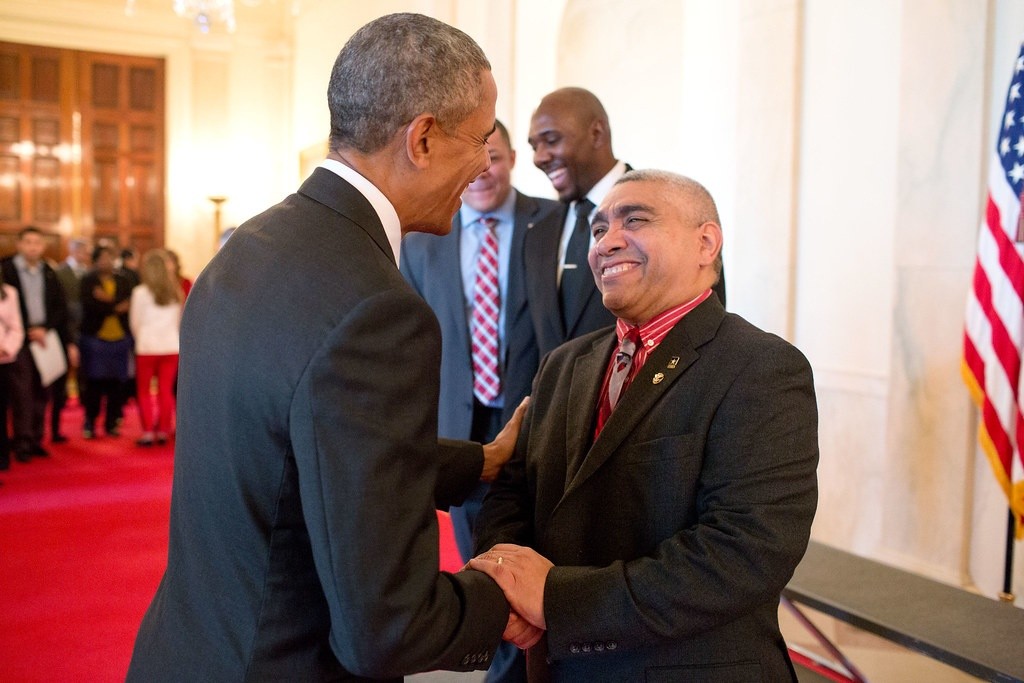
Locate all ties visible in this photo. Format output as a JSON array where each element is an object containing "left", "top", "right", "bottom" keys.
[
  {"left": 470, "top": 217, "right": 501, "bottom": 400},
  {"left": 558, "top": 199, "right": 597, "bottom": 336},
  {"left": 588, "top": 328, "right": 640, "bottom": 445}
]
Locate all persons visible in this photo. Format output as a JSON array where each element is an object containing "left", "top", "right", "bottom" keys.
[
  {"left": 522, "top": 87, "right": 726, "bottom": 366},
  {"left": 399, "top": 118, "right": 559, "bottom": 683},
  {"left": 0, "top": 227, "right": 193, "bottom": 473},
  {"left": 460, "top": 169, "right": 820, "bottom": 683},
  {"left": 121, "top": 14, "right": 531, "bottom": 683}
]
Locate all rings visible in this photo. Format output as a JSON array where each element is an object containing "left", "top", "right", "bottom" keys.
[{"left": 497, "top": 556, "right": 504, "bottom": 565}]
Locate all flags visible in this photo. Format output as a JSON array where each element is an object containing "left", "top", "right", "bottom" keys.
[{"left": 956, "top": 39, "right": 1024, "bottom": 545}]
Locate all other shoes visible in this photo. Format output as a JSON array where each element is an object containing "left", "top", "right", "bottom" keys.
[
  {"left": 83, "top": 426, "right": 96, "bottom": 443},
  {"left": 12, "top": 443, "right": 49, "bottom": 461},
  {"left": 108, "top": 427, "right": 120, "bottom": 437},
  {"left": 54, "top": 437, "right": 65, "bottom": 442},
  {"left": 136, "top": 438, "right": 154, "bottom": 447},
  {"left": 161, "top": 436, "right": 174, "bottom": 449}
]
